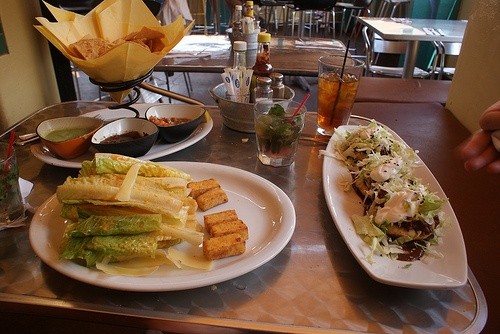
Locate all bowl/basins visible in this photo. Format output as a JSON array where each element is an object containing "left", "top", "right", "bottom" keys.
[
  {"left": 207, "top": 82, "right": 295, "bottom": 134},
  {"left": 91, "top": 118, "right": 158, "bottom": 158},
  {"left": 36, "top": 117, "right": 104, "bottom": 160},
  {"left": 145, "top": 105, "right": 206, "bottom": 144}
]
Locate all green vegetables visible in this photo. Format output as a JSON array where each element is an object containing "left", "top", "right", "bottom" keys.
[
  {"left": 263, "top": 103, "right": 303, "bottom": 155},
  {"left": 0, "top": 159, "right": 21, "bottom": 216}
]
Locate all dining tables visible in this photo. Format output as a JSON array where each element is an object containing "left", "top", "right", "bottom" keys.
[
  {"left": 0, "top": 100, "right": 488, "bottom": 334},
  {"left": 356, "top": 17, "right": 469, "bottom": 81},
  {"left": 154, "top": 35, "right": 353, "bottom": 111}
]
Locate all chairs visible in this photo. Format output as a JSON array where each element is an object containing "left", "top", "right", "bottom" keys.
[
  {"left": 437, "top": 41, "right": 462, "bottom": 81},
  {"left": 362, "top": 25, "right": 439, "bottom": 80},
  {"left": 254, "top": 0, "right": 373, "bottom": 37}
]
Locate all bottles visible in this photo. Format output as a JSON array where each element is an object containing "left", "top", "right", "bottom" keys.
[
  {"left": 230, "top": 33, "right": 285, "bottom": 102},
  {"left": 231, "top": 1, "right": 260, "bottom": 34}
]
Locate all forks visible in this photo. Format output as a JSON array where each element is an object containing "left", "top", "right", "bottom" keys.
[{"left": 19, "top": 114, "right": 104, "bottom": 140}]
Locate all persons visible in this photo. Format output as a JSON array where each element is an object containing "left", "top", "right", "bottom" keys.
[
  {"left": 462, "top": 102, "right": 500, "bottom": 174},
  {"left": 157, "top": 0, "right": 192, "bottom": 35}
]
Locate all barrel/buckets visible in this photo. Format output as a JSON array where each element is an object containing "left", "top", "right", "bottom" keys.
[{"left": 225, "top": 27, "right": 267, "bottom": 52}]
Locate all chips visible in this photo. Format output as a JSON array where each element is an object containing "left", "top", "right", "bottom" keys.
[{"left": 72, "top": 25, "right": 166, "bottom": 61}]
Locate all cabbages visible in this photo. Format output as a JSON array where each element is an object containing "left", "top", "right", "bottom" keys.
[
  {"left": 318, "top": 118, "right": 449, "bottom": 269},
  {"left": 56, "top": 153, "right": 204, "bottom": 268}
]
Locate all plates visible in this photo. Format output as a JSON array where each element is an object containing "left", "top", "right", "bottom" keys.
[
  {"left": 323, "top": 125, "right": 468, "bottom": 288},
  {"left": 31, "top": 103, "right": 214, "bottom": 168},
  {"left": 29, "top": 161, "right": 295, "bottom": 291}
]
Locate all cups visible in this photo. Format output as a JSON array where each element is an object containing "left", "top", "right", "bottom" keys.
[
  {"left": 253, "top": 97, "right": 308, "bottom": 166},
  {"left": 318, "top": 56, "right": 365, "bottom": 137},
  {"left": 0, "top": 142, "right": 25, "bottom": 226}
]
